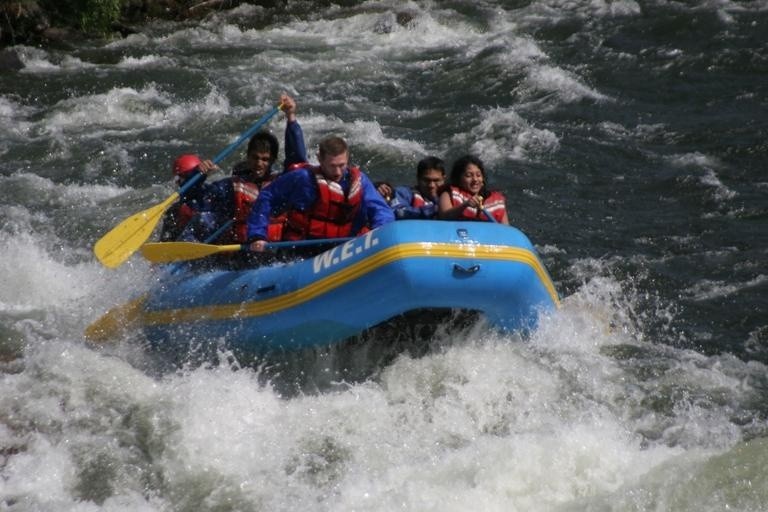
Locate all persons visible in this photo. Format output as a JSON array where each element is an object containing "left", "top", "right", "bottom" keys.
[
  {"left": 247, "top": 137, "right": 396, "bottom": 254},
  {"left": 371, "top": 177, "right": 395, "bottom": 209},
  {"left": 392, "top": 153, "right": 447, "bottom": 216},
  {"left": 160, "top": 152, "right": 214, "bottom": 247},
  {"left": 181, "top": 94, "right": 306, "bottom": 276},
  {"left": 437, "top": 154, "right": 508, "bottom": 222}
]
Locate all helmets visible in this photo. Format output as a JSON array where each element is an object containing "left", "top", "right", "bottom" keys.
[{"left": 174, "top": 154, "right": 201, "bottom": 174}]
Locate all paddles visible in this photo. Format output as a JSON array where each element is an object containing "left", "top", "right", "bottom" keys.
[
  {"left": 84, "top": 220, "right": 233, "bottom": 354},
  {"left": 95, "top": 102, "right": 283, "bottom": 270},
  {"left": 138, "top": 238, "right": 352, "bottom": 262}
]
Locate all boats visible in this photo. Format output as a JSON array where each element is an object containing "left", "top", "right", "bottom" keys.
[{"left": 142, "top": 220, "right": 562, "bottom": 357}]
